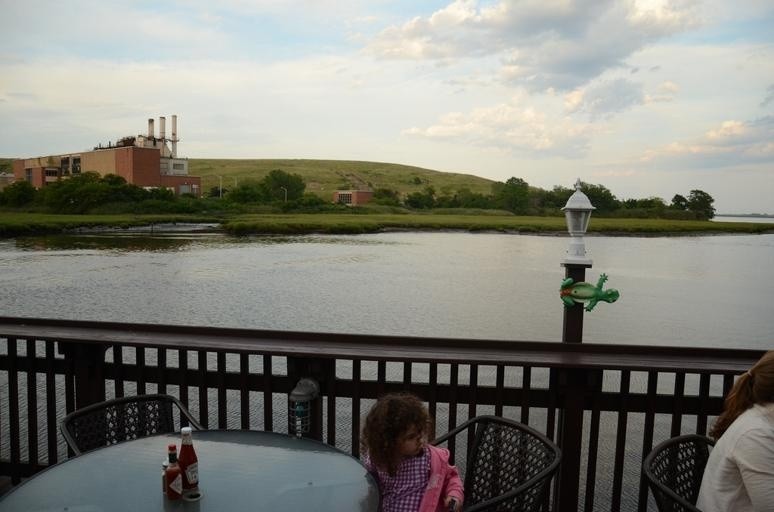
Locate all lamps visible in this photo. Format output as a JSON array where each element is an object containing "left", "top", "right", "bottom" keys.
[{"left": 289, "top": 378, "right": 318, "bottom": 433}]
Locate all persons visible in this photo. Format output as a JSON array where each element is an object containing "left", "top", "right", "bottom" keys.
[
  {"left": 361, "top": 394, "right": 463, "bottom": 512},
  {"left": 694, "top": 349, "right": 774, "bottom": 512}
]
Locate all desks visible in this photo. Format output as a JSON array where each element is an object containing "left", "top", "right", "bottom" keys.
[{"left": 0, "top": 428, "right": 383, "bottom": 511}]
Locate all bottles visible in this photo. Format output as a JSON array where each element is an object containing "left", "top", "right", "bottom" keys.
[
  {"left": 165, "top": 444, "right": 183, "bottom": 502},
  {"left": 178, "top": 427, "right": 199, "bottom": 491}
]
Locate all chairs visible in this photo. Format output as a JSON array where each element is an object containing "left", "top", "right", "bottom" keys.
[
  {"left": 641, "top": 432, "right": 717, "bottom": 511},
  {"left": 59, "top": 392, "right": 205, "bottom": 457},
  {"left": 429, "top": 415, "right": 562, "bottom": 511}
]
